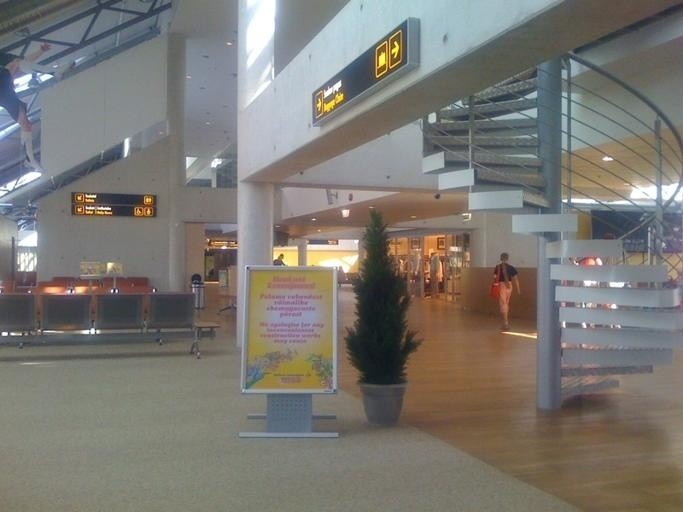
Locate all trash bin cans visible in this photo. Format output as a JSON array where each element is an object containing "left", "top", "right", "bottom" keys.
[{"left": 192, "top": 282, "right": 205, "bottom": 309}]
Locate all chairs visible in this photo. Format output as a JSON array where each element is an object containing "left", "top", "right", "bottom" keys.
[{"left": 0, "top": 277, "right": 200, "bottom": 363}]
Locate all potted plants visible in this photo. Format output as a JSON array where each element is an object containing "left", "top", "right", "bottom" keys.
[{"left": 345, "top": 207, "right": 423, "bottom": 427}]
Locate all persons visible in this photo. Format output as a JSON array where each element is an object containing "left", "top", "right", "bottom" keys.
[
  {"left": 413, "top": 251, "right": 421, "bottom": 299},
  {"left": 271, "top": 254, "right": 286, "bottom": 265},
  {"left": 428, "top": 252, "right": 441, "bottom": 298},
  {"left": 0, "top": 39, "right": 76, "bottom": 176},
  {"left": 492, "top": 252, "right": 521, "bottom": 332}
]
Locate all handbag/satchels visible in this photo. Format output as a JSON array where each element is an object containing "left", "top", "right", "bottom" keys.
[{"left": 489, "top": 263, "right": 500, "bottom": 298}]
